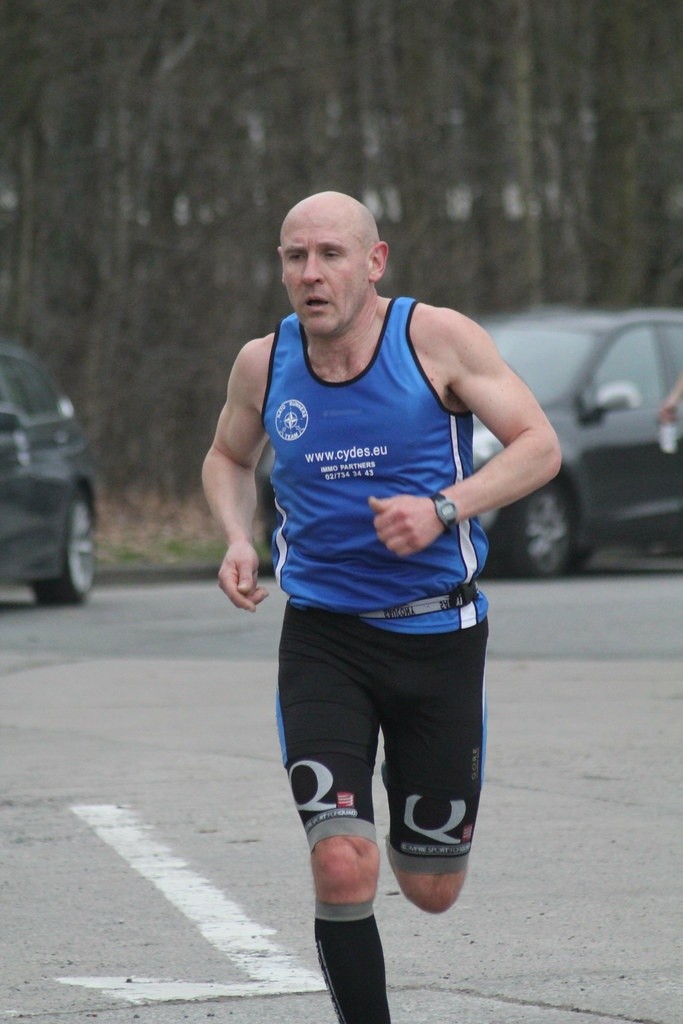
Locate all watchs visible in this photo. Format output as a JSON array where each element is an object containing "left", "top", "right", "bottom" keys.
[{"left": 429, "top": 491, "right": 456, "bottom": 536}]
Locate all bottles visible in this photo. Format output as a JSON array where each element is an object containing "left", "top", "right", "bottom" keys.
[{"left": 658, "top": 407, "right": 679, "bottom": 454}]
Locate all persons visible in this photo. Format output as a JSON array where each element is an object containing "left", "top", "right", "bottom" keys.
[
  {"left": 659, "top": 380, "right": 683, "bottom": 424},
  {"left": 202, "top": 190, "right": 560, "bottom": 1024}
]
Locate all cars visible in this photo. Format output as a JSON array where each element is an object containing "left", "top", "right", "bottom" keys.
[
  {"left": 1, "top": 350, "right": 104, "bottom": 611},
  {"left": 472, "top": 304, "right": 682, "bottom": 582}
]
